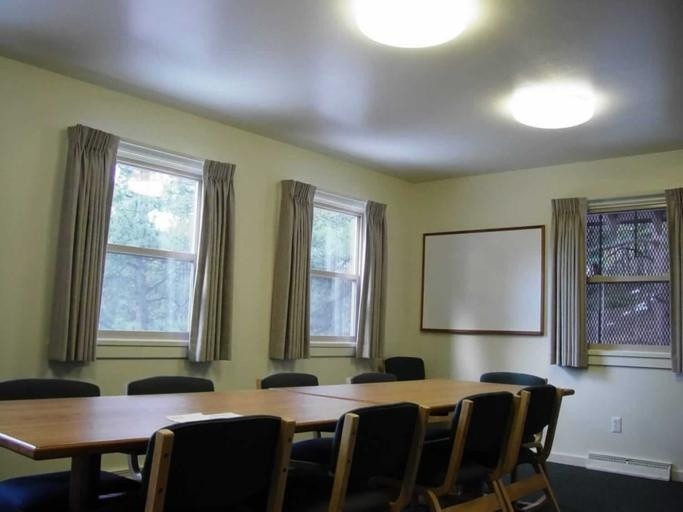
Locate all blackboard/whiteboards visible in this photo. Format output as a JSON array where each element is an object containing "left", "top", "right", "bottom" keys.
[{"left": 420, "top": 225, "right": 543, "bottom": 334}]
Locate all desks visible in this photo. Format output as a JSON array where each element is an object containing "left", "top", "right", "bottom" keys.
[{"left": 1, "top": 378, "right": 575, "bottom": 509}]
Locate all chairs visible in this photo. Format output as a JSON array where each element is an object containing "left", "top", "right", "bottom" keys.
[
  {"left": 126, "top": 375, "right": 217, "bottom": 478},
  {"left": 256, "top": 373, "right": 334, "bottom": 475},
  {"left": 0, "top": 378, "right": 141, "bottom": 512},
  {"left": 384, "top": 357, "right": 427, "bottom": 381},
  {"left": 415, "top": 389, "right": 521, "bottom": 512},
  {"left": 348, "top": 372, "right": 400, "bottom": 384},
  {"left": 480, "top": 372, "right": 548, "bottom": 454},
  {"left": 498, "top": 384, "right": 562, "bottom": 512},
  {"left": 287, "top": 402, "right": 430, "bottom": 512},
  {"left": 95, "top": 414, "right": 297, "bottom": 512}
]
